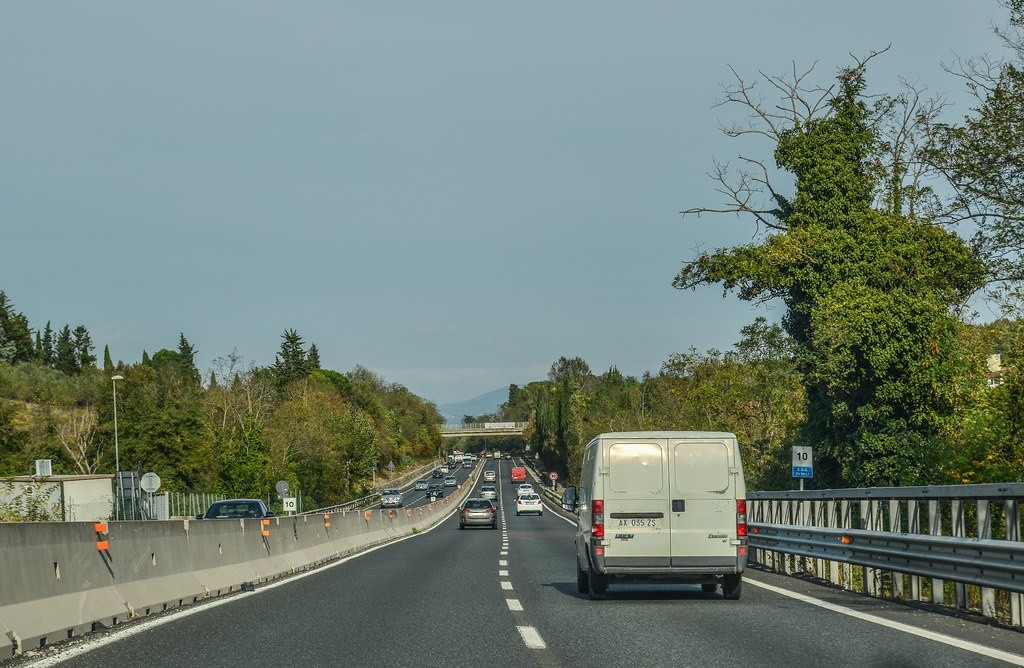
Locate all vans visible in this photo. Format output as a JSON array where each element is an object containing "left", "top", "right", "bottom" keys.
[{"left": 563, "top": 430, "right": 750, "bottom": 599}]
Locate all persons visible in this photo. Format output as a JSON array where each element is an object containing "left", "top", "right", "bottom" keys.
[{"left": 247, "top": 506, "right": 258, "bottom": 516}]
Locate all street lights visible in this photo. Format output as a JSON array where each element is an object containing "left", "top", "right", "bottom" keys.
[{"left": 112, "top": 374, "right": 124, "bottom": 471}]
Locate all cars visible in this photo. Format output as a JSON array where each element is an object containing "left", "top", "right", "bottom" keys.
[{"left": 195, "top": 449, "right": 544, "bottom": 529}]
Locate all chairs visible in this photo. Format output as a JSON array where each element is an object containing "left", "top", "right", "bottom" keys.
[
  {"left": 468, "top": 503, "right": 474, "bottom": 508},
  {"left": 479, "top": 504, "right": 485, "bottom": 507},
  {"left": 216, "top": 507, "right": 230, "bottom": 518}
]
